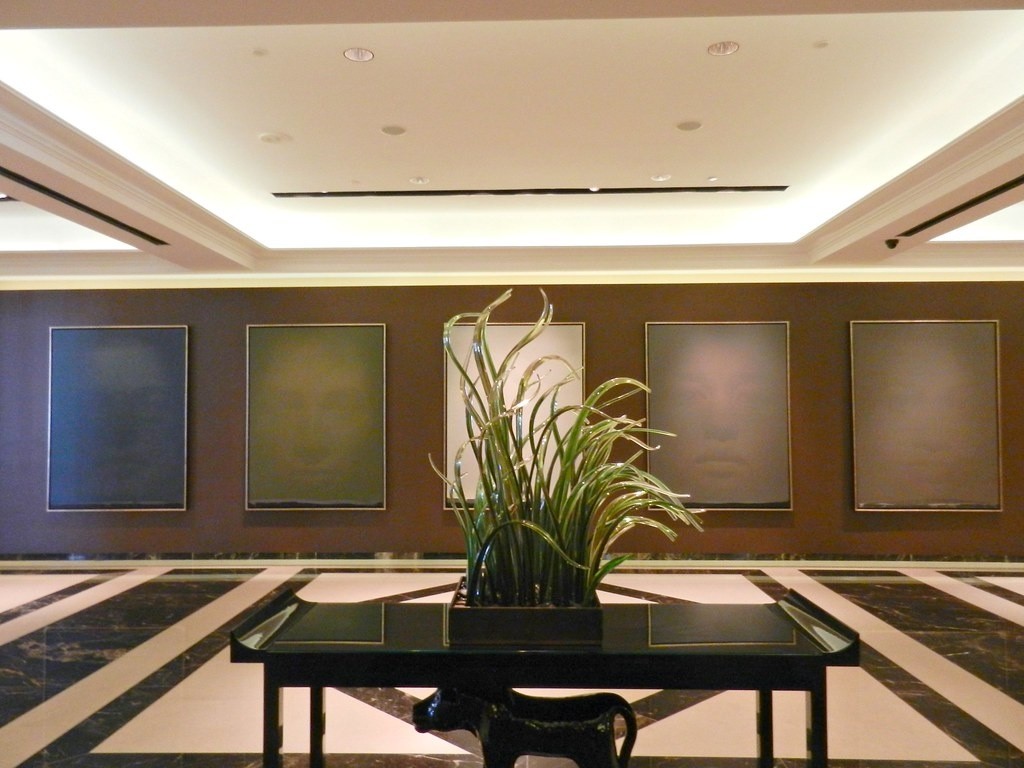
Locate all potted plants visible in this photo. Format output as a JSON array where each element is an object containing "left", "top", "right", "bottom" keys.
[{"left": 427, "top": 285, "right": 704, "bottom": 645}]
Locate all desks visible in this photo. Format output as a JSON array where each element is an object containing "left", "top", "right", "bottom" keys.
[{"left": 230, "top": 587, "right": 862, "bottom": 768}]
